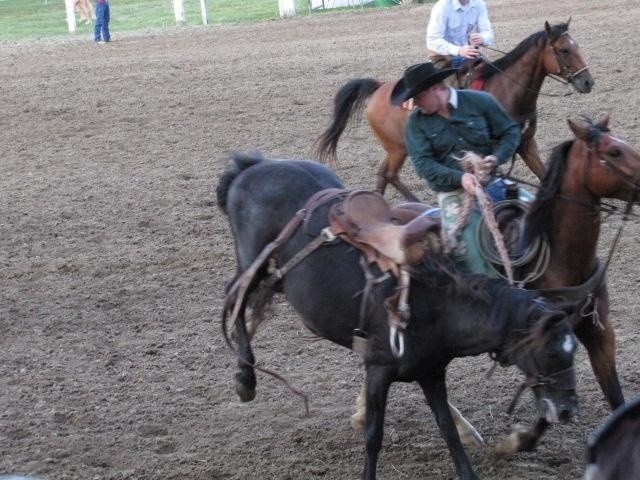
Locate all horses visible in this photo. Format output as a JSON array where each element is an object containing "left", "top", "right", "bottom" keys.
[
  {"left": 211, "top": 151, "right": 595, "bottom": 480},
  {"left": 351, "top": 106, "right": 640, "bottom": 456},
  {"left": 308, "top": 13, "right": 598, "bottom": 203}
]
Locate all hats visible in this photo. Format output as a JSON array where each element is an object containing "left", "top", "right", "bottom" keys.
[{"left": 391, "top": 61, "right": 460, "bottom": 105}]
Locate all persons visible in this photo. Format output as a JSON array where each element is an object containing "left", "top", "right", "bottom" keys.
[
  {"left": 425, "top": 0, "right": 496, "bottom": 89},
  {"left": 94, "top": 0, "right": 110, "bottom": 42},
  {"left": 390, "top": 62, "right": 536, "bottom": 280}
]
